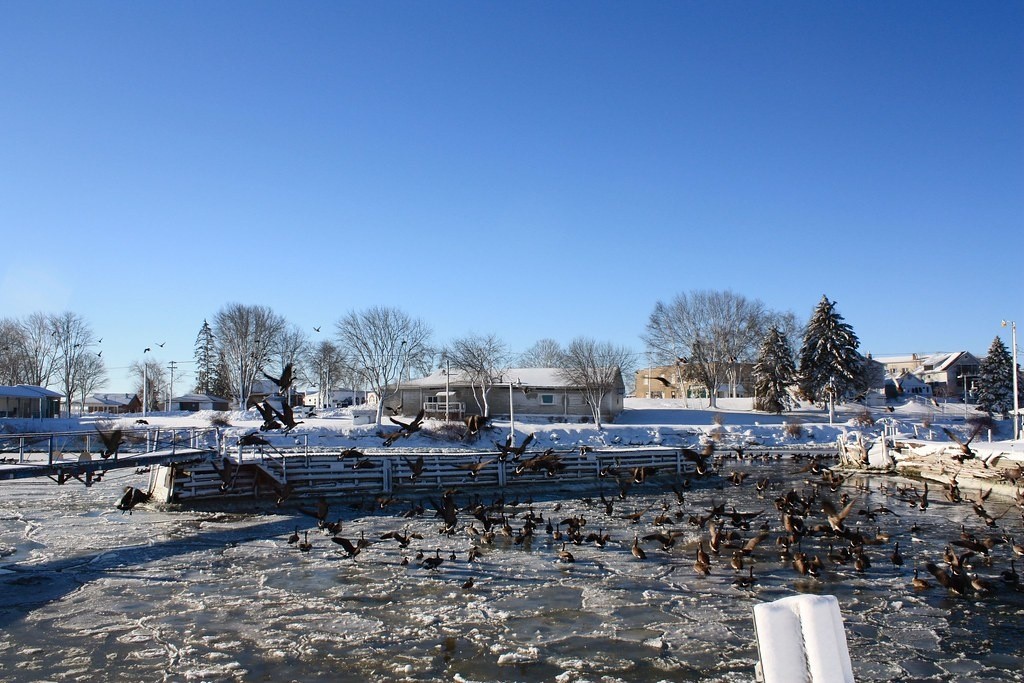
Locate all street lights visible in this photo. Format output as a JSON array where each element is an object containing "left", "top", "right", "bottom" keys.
[
  {"left": 508, "top": 377, "right": 523, "bottom": 447},
  {"left": 1000, "top": 319, "right": 1019, "bottom": 440}
]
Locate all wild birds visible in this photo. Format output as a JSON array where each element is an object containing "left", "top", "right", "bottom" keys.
[{"left": 0, "top": 326, "right": 1024, "bottom": 597}]
